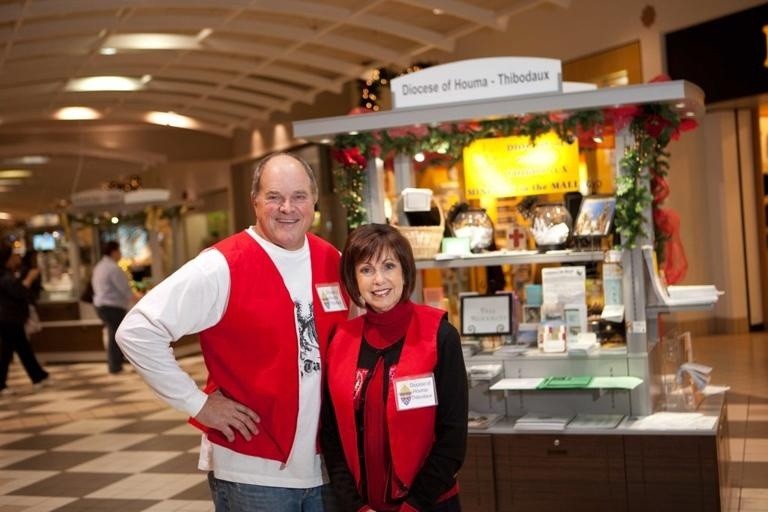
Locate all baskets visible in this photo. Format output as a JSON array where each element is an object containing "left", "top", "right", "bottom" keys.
[{"left": 391, "top": 190, "right": 445, "bottom": 260}]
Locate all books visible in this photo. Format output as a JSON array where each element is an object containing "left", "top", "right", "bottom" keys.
[
  {"left": 667, "top": 283, "right": 721, "bottom": 307},
  {"left": 642, "top": 245, "right": 668, "bottom": 305},
  {"left": 512, "top": 412, "right": 576, "bottom": 430}
]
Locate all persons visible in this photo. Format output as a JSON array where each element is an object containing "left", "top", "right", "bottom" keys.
[
  {"left": 0, "top": 245, "right": 56, "bottom": 396},
  {"left": 319, "top": 224, "right": 468, "bottom": 512},
  {"left": 91, "top": 239, "right": 138, "bottom": 376},
  {"left": 114, "top": 153, "right": 366, "bottom": 512}
]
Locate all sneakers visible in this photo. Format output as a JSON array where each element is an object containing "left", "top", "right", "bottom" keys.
[
  {"left": 1, "top": 387, "right": 17, "bottom": 396},
  {"left": 34, "top": 377, "right": 57, "bottom": 388}
]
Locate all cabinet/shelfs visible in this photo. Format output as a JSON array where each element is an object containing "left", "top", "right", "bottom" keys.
[{"left": 291, "top": 80, "right": 726, "bottom": 510}]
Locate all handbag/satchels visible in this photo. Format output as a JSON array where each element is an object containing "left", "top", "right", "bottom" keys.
[{"left": 80, "top": 280, "right": 93, "bottom": 303}]
